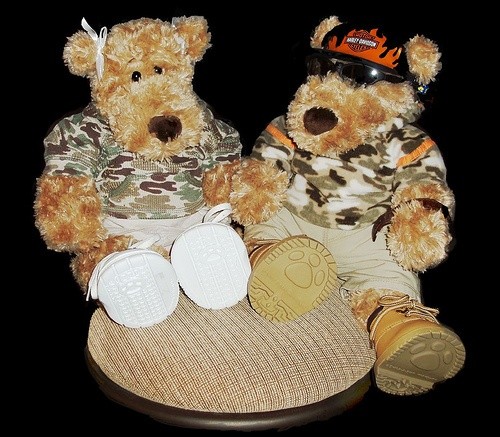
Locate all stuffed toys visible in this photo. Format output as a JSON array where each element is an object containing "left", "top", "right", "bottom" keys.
[
  {"left": 34, "top": 15, "right": 252, "bottom": 329},
  {"left": 230, "top": 17, "right": 466, "bottom": 397}
]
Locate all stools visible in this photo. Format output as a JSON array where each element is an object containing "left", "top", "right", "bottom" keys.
[{"left": 83, "top": 278, "right": 379, "bottom": 432}]
